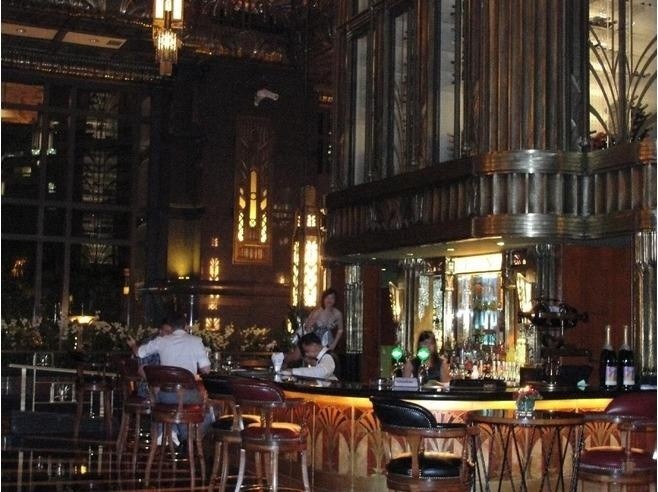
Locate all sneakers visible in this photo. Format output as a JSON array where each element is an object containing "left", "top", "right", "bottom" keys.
[
  {"left": 170, "top": 430, "right": 181, "bottom": 447},
  {"left": 156, "top": 432, "right": 169, "bottom": 446}
]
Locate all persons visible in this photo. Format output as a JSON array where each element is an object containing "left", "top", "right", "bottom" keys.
[
  {"left": 281, "top": 332, "right": 342, "bottom": 381},
  {"left": 126, "top": 313, "right": 214, "bottom": 445},
  {"left": 402, "top": 329, "right": 451, "bottom": 382},
  {"left": 303, "top": 287, "right": 345, "bottom": 350},
  {"left": 134, "top": 321, "right": 181, "bottom": 447}
]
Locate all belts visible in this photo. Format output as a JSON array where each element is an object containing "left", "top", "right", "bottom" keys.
[{"left": 160, "top": 387, "right": 194, "bottom": 393}]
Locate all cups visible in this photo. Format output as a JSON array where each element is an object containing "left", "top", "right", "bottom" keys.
[
  {"left": 440, "top": 343, "right": 518, "bottom": 382},
  {"left": 208, "top": 351, "right": 222, "bottom": 372},
  {"left": 542, "top": 354, "right": 560, "bottom": 387}
]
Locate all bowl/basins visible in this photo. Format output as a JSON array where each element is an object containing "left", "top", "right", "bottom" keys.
[{"left": 367, "top": 376, "right": 395, "bottom": 390}]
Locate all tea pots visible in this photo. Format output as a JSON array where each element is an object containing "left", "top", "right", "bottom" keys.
[{"left": 222, "top": 356, "right": 239, "bottom": 370}]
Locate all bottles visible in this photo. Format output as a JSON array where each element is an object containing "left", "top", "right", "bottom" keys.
[
  {"left": 616, "top": 325, "right": 635, "bottom": 392},
  {"left": 597, "top": 325, "right": 619, "bottom": 392}
]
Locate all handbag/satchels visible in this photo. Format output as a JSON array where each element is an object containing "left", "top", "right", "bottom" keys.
[{"left": 211, "top": 416, "right": 258, "bottom": 431}]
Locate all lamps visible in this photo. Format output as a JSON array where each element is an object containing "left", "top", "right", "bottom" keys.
[{"left": 152, "top": 0, "right": 185, "bottom": 77}]
